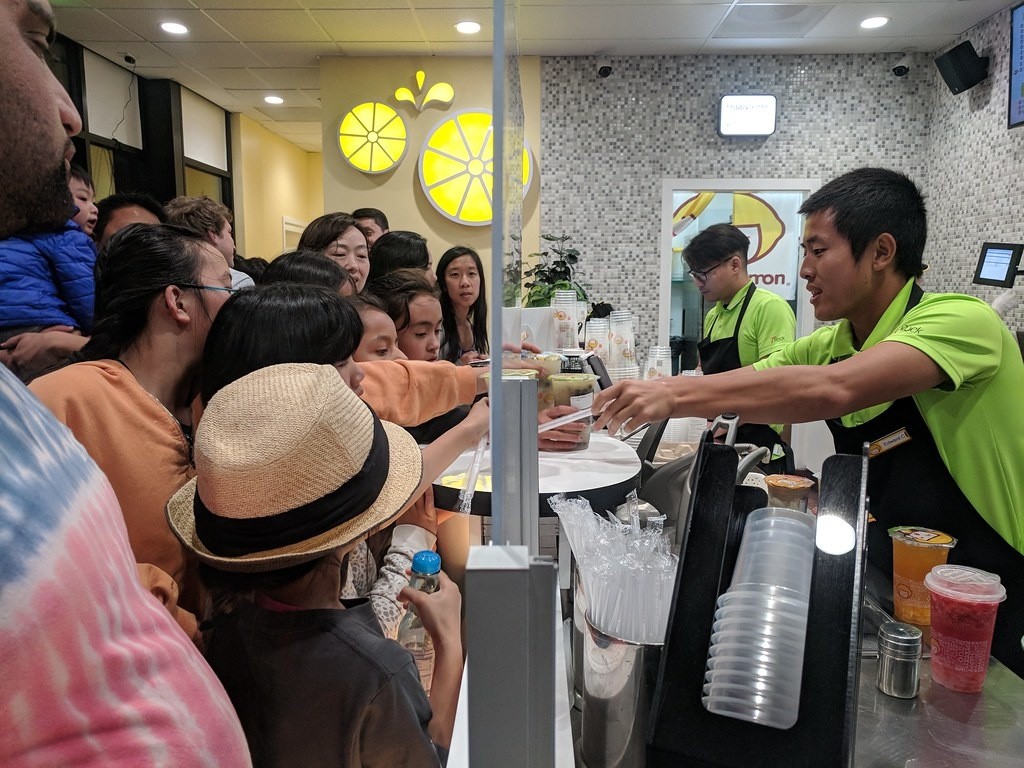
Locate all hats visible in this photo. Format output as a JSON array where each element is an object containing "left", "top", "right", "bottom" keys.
[{"left": 164, "top": 361, "right": 422, "bottom": 570}]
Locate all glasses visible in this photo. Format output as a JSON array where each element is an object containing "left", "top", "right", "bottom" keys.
[
  {"left": 147, "top": 282, "right": 238, "bottom": 299},
  {"left": 688, "top": 255, "right": 739, "bottom": 280}
]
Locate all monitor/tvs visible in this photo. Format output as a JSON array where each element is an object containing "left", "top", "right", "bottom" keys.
[
  {"left": 1007, "top": 1, "right": 1024, "bottom": 130},
  {"left": 973, "top": 242, "right": 1024, "bottom": 289}
]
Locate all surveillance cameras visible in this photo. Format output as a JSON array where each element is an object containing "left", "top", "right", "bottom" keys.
[
  {"left": 892, "top": 55, "right": 914, "bottom": 77},
  {"left": 596, "top": 59, "right": 612, "bottom": 78}
]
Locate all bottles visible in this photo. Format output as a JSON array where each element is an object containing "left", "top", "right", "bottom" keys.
[
  {"left": 397, "top": 550, "right": 441, "bottom": 698},
  {"left": 876, "top": 623, "right": 922, "bottom": 697}
]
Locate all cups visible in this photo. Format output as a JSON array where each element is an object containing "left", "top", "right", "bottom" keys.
[
  {"left": 887, "top": 526, "right": 958, "bottom": 626},
  {"left": 551, "top": 290, "right": 708, "bottom": 451},
  {"left": 701, "top": 507, "right": 817, "bottom": 729},
  {"left": 570, "top": 563, "right": 666, "bottom": 768},
  {"left": 480, "top": 369, "right": 540, "bottom": 389},
  {"left": 547, "top": 373, "right": 601, "bottom": 450},
  {"left": 763, "top": 473, "right": 815, "bottom": 513},
  {"left": 923, "top": 566, "right": 1007, "bottom": 693}
]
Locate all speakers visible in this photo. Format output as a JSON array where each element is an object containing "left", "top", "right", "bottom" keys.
[{"left": 935, "top": 40, "right": 988, "bottom": 95}]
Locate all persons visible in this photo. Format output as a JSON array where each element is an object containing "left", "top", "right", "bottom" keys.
[
  {"left": 680, "top": 224, "right": 797, "bottom": 474},
  {"left": 182, "top": 282, "right": 442, "bottom": 641},
  {"left": 376, "top": 266, "right": 470, "bottom": 585},
  {"left": 0, "top": 0, "right": 257, "bottom": 768},
  {"left": 365, "top": 230, "right": 429, "bottom": 292},
  {"left": 353, "top": 208, "right": 390, "bottom": 250},
  {"left": 589, "top": 166, "right": 1024, "bottom": 678},
  {"left": 163, "top": 361, "right": 491, "bottom": 768},
  {"left": 17, "top": 218, "right": 551, "bottom": 647},
  {"left": 340, "top": 294, "right": 408, "bottom": 362},
  {"left": 265, "top": 250, "right": 357, "bottom": 298},
  {"left": 296, "top": 213, "right": 369, "bottom": 292},
  {"left": 0, "top": 164, "right": 273, "bottom": 384},
  {"left": 432, "top": 243, "right": 487, "bottom": 366}
]
